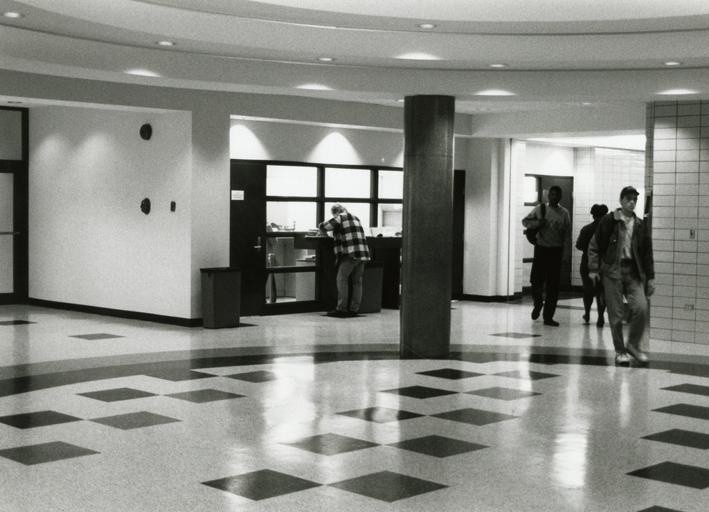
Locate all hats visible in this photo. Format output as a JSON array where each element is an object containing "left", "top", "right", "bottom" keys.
[{"left": 619, "top": 186, "right": 640, "bottom": 200}]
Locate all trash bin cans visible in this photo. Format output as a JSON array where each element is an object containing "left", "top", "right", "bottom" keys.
[
  {"left": 358, "top": 257, "right": 385, "bottom": 314},
  {"left": 200, "top": 267, "right": 244, "bottom": 329}
]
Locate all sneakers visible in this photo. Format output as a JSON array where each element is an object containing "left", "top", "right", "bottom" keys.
[
  {"left": 327, "top": 309, "right": 357, "bottom": 318},
  {"left": 530, "top": 307, "right": 604, "bottom": 328},
  {"left": 625, "top": 337, "right": 649, "bottom": 365},
  {"left": 613, "top": 348, "right": 630, "bottom": 366}
]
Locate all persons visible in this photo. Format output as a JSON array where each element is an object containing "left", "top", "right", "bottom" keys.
[
  {"left": 576, "top": 203, "right": 609, "bottom": 328},
  {"left": 521, "top": 185, "right": 573, "bottom": 328},
  {"left": 318, "top": 204, "right": 372, "bottom": 319},
  {"left": 587, "top": 186, "right": 656, "bottom": 369}
]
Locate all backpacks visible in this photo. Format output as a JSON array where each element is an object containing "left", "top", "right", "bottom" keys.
[{"left": 521, "top": 205, "right": 547, "bottom": 244}]
